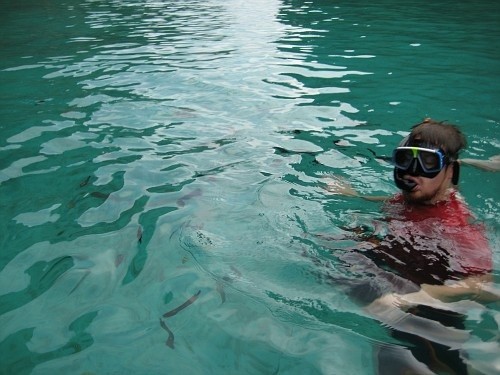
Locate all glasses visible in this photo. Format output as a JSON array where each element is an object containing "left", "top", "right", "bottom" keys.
[{"left": 392, "top": 136, "right": 453, "bottom": 179}]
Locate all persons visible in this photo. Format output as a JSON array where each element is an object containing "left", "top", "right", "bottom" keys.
[{"left": 325, "top": 118, "right": 494, "bottom": 375}]
